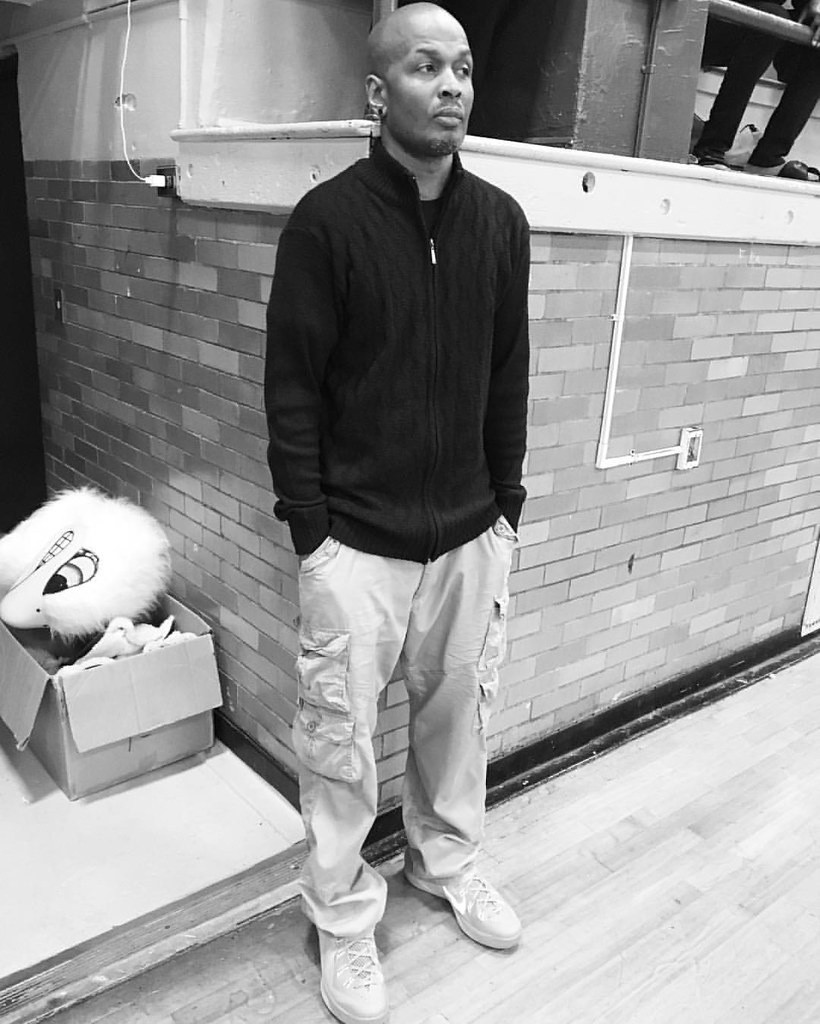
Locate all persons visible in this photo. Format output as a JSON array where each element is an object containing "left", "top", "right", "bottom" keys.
[
  {"left": 691, "top": 0, "right": 820, "bottom": 182},
  {"left": 264, "top": 2, "right": 530, "bottom": 1024}
]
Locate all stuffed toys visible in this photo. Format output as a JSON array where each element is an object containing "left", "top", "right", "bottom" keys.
[{"left": 0, "top": 485, "right": 171, "bottom": 645}]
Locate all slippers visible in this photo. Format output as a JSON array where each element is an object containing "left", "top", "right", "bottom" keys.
[
  {"left": 699, "top": 154, "right": 731, "bottom": 171},
  {"left": 778, "top": 160, "right": 820, "bottom": 183}
]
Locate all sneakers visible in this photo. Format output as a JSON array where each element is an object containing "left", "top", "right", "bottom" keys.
[
  {"left": 317, "top": 926, "right": 387, "bottom": 1024},
  {"left": 405, "top": 869, "right": 520, "bottom": 948}
]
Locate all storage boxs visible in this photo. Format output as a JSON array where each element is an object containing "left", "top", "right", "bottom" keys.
[{"left": 1, "top": 593, "right": 224, "bottom": 805}]
